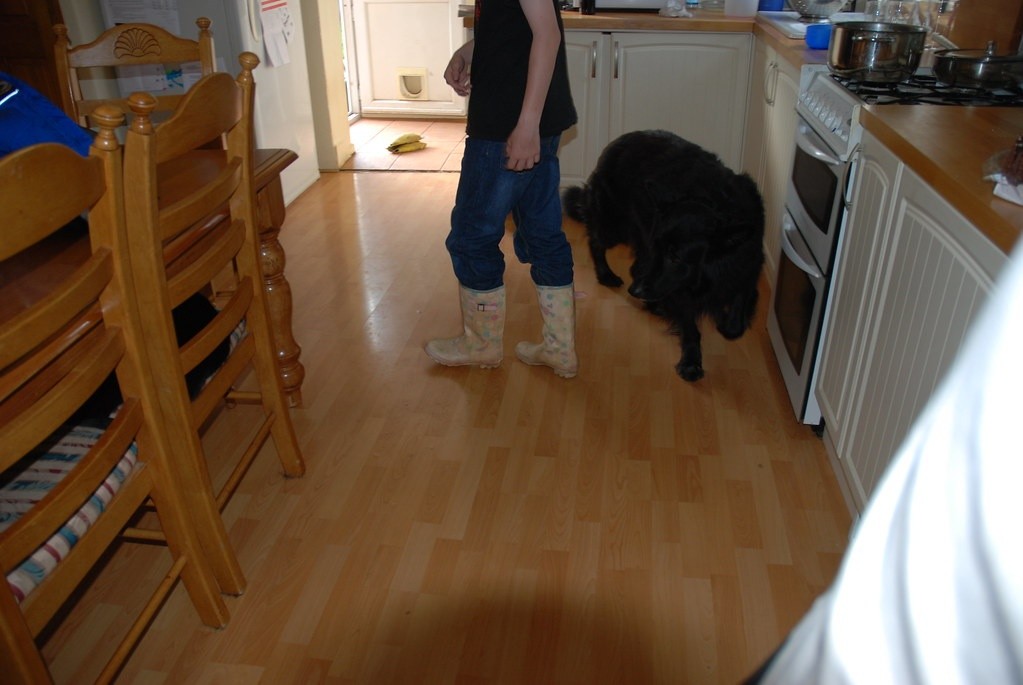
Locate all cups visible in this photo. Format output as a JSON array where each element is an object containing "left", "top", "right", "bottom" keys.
[
  {"left": 865, "top": 0, "right": 920, "bottom": 24},
  {"left": 579, "top": 0, "right": 595, "bottom": 15}
]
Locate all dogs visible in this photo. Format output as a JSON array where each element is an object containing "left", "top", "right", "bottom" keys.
[{"left": 561, "top": 129, "right": 765, "bottom": 382}]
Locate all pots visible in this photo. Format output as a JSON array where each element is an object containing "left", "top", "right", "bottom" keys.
[
  {"left": 931, "top": 40, "right": 1023, "bottom": 90},
  {"left": 826, "top": 21, "right": 929, "bottom": 84}
]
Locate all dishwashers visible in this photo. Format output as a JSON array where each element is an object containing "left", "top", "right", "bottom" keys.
[{"left": 765, "top": 111, "right": 860, "bottom": 426}]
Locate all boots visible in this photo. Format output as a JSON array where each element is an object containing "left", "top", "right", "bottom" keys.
[
  {"left": 515, "top": 282, "right": 579, "bottom": 378},
  {"left": 425, "top": 284, "right": 506, "bottom": 370}
]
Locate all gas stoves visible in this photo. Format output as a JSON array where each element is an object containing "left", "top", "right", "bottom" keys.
[{"left": 797, "top": 63, "right": 1023, "bottom": 163}]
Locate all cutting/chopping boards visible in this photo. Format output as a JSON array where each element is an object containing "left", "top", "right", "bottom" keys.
[{"left": 757, "top": 11, "right": 872, "bottom": 39}]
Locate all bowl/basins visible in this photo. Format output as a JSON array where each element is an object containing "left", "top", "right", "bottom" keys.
[
  {"left": 788, "top": 0, "right": 848, "bottom": 23},
  {"left": 757, "top": 0, "right": 785, "bottom": 12},
  {"left": 805, "top": 24, "right": 832, "bottom": 48},
  {"left": 723, "top": 0, "right": 759, "bottom": 19}
]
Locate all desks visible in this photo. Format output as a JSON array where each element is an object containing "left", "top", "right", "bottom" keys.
[{"left": 0, "top": 149, "right": 303, "bottom": 408}]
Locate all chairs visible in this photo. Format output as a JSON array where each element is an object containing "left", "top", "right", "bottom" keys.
[
  {"left": 83, "top": 52, "right": 308, "bottom": 601},
  {"left": 52, "top": 16, "right": 230, "bottom": 153},
  {"left": 0, "top": 104, "right": 233, "bottom": 685}
]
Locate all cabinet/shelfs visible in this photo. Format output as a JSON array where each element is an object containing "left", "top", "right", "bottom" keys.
[{"left": 462, "top": 7, "right": 1023, "bottom": 541}]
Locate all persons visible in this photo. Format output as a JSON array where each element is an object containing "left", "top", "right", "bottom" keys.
[
  {"left": 737, "top": 233, "right": 1023, "bottom": 684},
  {"left": 424, "top": 0, "right": 581, "bottom": 379}
]
